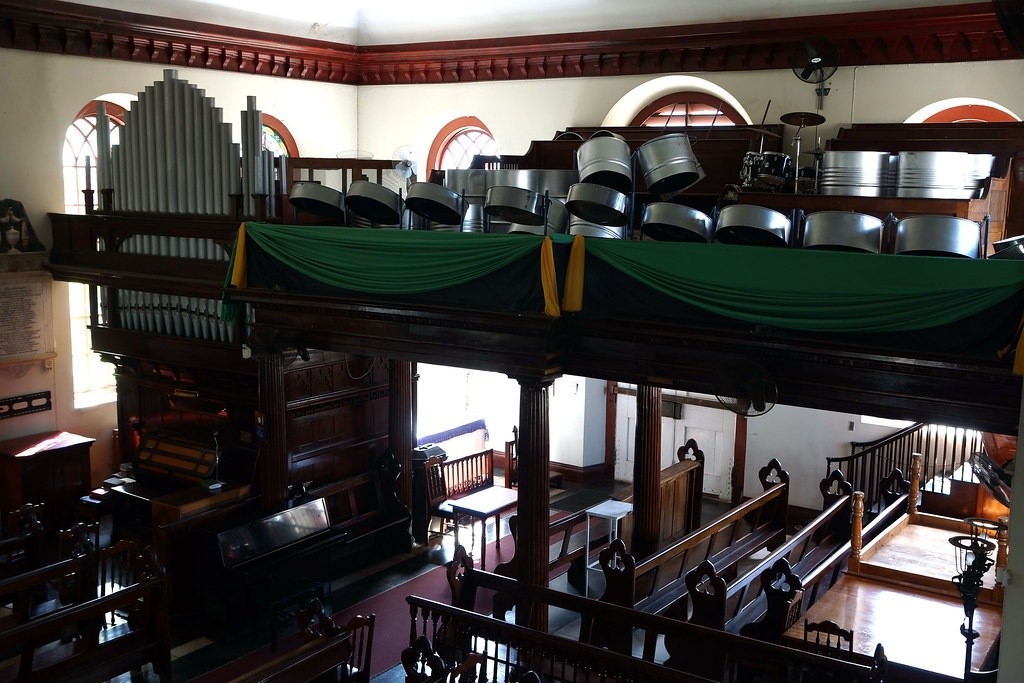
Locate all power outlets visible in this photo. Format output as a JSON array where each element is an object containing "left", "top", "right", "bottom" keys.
[{"left": 44, "top": 359, "right": 55, "bottom": 372}]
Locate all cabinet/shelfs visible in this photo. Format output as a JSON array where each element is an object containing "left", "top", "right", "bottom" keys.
[{"left": 0, "top": 430, "right": 97, "bottom": 540}]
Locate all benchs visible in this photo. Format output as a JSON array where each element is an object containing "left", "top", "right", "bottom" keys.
[
  {"left": 468, "top": 124, "right": 786, "bottom": 192},
  {"left": 399, "top": 440, "right": 911, "bottom": 683},
  {"left": 0, "top": 499, "right": 375, "bottom": 682},
  {"left": 823, "top": 120, "right": 1024, "bottom": 257}
]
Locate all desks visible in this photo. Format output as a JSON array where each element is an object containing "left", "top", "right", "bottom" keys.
[
  {"left": 586, "top": 500, "right": 633, "bottom": 596},
  {"left": 448, "top": 486, "right": 517, "bottom": 570}
]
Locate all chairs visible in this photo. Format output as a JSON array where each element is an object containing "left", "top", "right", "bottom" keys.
[{"left": 419, "top": 455, "right": 474, "bottom": 550}]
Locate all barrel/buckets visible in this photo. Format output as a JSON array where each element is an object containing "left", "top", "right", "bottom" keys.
[{"left": 428, "top": 129, "right": 1024, "bottom": 259}]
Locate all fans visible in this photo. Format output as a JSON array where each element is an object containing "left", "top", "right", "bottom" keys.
[
  {"left": 790, "top": 36, "right": 841, "bottom": 110},
  {"left": 714, "top": 395, "right": 777, "bottom": 418}
]
